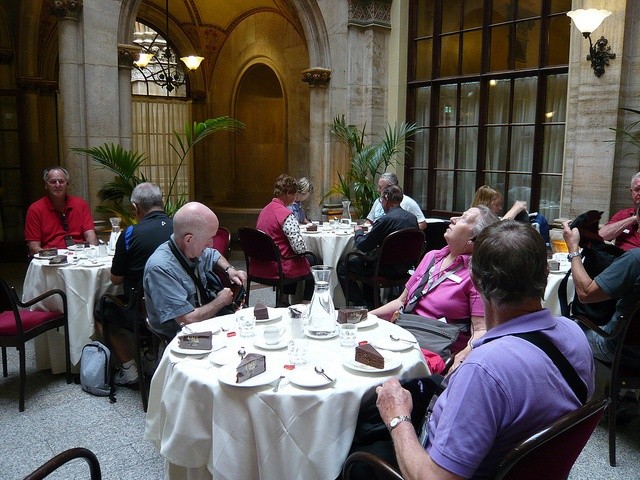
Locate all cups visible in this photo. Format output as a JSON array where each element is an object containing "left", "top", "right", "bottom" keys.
[
  {"left": 237, "top": 314, "right": 256, "bottom": 337},
  {"left": 98, "top": 244, "right": 107, "bottom": 256},
  {"left": 329, "top": 220, "right": 334, "bottom": 224},
  {"left": 288, "top": 340, "right": 310, "bottom": 364},
  {"left": 338, "top": 324, "right": 357, "bottom": 347},
  {"left": 263, "top": 326, "right": 286, "bottom": 344},
  {"left": 313, "top": 221, "right": 319, "bottom": 228},
  {"left": 351, "top": 222, "right": 357, "bottom": 228}
]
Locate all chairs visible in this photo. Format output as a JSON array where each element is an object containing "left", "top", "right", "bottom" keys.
[
  {"left": 1, "top": 278, "right": 73, "bottom": 411},
  {"left": 212, "top": 226, "right": 232, "bottom": 260},
  {"left": 23, "top": 447, "right": 104, "bottom": 479},
  {"left": 239, "top": 227, "right": 318, "bottom": 308},
  {"left": 567, "top": 291, "right": 640, "bottom": 466},
  {"left": 424, "top": 222, "right": 451, "bottom": 254},
  {"left": 343, "top": 227, "right": 427, "bottom": 309},
  {"left": 100, "top": 277, "right": 167, "bottom": 412},
  {"left": 340, "top": 397, "right": 613, "bottom": 480},
  {"left": 145, "top": 264, "right": 244, "bottom": 363}
]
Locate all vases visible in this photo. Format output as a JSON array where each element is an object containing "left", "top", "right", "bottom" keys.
[{"left": 92, "top": 220, "right": 107, "bottom": 233}]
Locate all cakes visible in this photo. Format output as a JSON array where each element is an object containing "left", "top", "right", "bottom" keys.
[
  {"left": 336, "top": 309, "right": 368, "bottom": 324},
  {"left": 39, "top": 248, "right": 57, "bottom": 257},
  {"left": 178, "top": 331, "right": 213, "bottom": 350},
  {"left": 254, "top": 303, "right": 269, "bottom": 320},
  {"left": 49, "top": 255, "right": 67, "bottom": 264},
  {"left": 547, "top": 261, "right": 560, "bottom": 271},
  {"left": 236, "top": 353, "right": 266, "bottom": 383},
  {"left": 362, "top": 227, "right": 368, "bottom": 231},
  {"left": 355, "top": 344, "right": 384, "bottom": 370},
  {"left": 306, "top": 225, "right": 317, "bottom": 231}
]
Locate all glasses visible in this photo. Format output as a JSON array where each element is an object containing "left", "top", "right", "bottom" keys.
[
  {"left": 378, "top": 193, "right": 387, "bottom": 203},
  {"left": 61, "top": 213, "right": 68, "bottom": 230},
  {"left": 289, "top": 308, "right": 301, "bottom": 318}
]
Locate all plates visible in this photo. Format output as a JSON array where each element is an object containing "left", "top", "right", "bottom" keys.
[
  {"left": 304, "top": 325, "right": 339, "bottom": 340},
  {"left": 289, "top": 302, "right": 310, "bottom": 319},
  {"left": 322, "top": 227, "right": 333, "bottom": 231},
  {"left": 182, "top": 321, "right": 222, "bottom": 334},
  {"left": 336, "top": 230, "right": 351, "bottom": 236},
  {"left": 81, "top": 260, "right": 104, "bottom": 267},
  {"left": 217, "top": 360, "right": 280, "bottom": 388},
  {"left": 336, "top": 313, "right": 378, "bottom": 329},
  {"left": 34, "top": 253, "right": 59, "bottom": 259},
  {"left": 375, "top": 333, "right": 414, "bottom": 351},
  {"left": 67, "top": 244, "right": 87, "bottom": 251},
  {"left": 288, "top": 366, "right": 336, "bottom": 387},
  {"left": 42, "top": 259, "right": 71, "bottom": 267},
  {"left": 209, "top": 346, "right": 251, "bottom": 365},
  {"left": 171, "top": 335, "right": 228, "bottom": 354},
  {"left": 301, "top": 230, "right": 321, "bottom": 233},
  {"left": 342, "top": 350, "right": 403, "bottom": 373},
  {"left": 253, "top": 331, "right": 292, "bottom": 351},
  {"left": 234, "top": 306, "right": 282, "bottom": 323}
]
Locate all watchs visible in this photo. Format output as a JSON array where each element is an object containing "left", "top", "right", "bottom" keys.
[
  {"left": 388, "top": 415, "right": 412, "bottom": 431},
  {"left": 568, "top": 252, "right": 582, "bottom": 260},
  {"left": 224, "top": 265, "right": 235, "bottom": 271}
]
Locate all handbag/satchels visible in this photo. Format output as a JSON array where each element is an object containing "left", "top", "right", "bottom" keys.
[
  {"left": 395, "top": 313, "right": 461, "bottom": 355},
  {"left": 168, "top": 239, "right": 245, "bottom": 316},
  {"left": 352, "top": 376, "right": 445, "bottom": 467}
]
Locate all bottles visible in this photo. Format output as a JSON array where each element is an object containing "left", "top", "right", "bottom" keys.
[
  {"left": 308, "top": 264, "right": 336, "bottom": 327},
  {"left": 107, "top": 217, "right": 124, "bottom": 255},
  {"left": 341, "top": 200, "right": 351, "bottom": 228}
]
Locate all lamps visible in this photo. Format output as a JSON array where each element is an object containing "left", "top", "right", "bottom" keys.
[
  {"left": 131, "top": 0, "right": 205, "bottom": 92},
  {"left": 566, "top": 8, "right": 617, "bottom": 78}
]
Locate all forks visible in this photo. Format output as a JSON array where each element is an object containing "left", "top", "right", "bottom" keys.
[{"left": 193, "top": 346, "right": 226, "bottom": 361}]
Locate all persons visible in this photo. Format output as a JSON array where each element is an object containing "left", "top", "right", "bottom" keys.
[
  {"left": 563, "top": 207, "right": 640, "bottom": 363},
  {"left": 364, "top": 173, "right": 427, "bottom": 231},
  {"left": 248, "top": 175, "right": 315, "bottom": 306},
  {"left": 598, "top": 172, "right": 640, "bottom": 252},
  {"left": 337, "top": 184, "right": 420, "bottom": 310},
  {"left": 101, "top": 183, "right": 174, "bottom": 387},
  {"left": 286, "top": 177, "right": 314, "bottom": 225},
  {"left": 375, "top": 220, "right": 597, "bottom": 479},
  {"left": 472, "top": 186, "right": 527, "bottom": 224},
  {"left": 143, "top": 202, "right": 248, "bottom": 337},
  {"left": 368, "top": 207, "right": 498, "bottom": 381},
  {"left": 25, "top": 167, "right": 98, "bottom": 253}
]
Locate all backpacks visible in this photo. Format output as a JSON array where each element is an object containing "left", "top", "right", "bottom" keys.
[
  {"left": 514, "top": 209, "right": 551, "bottom": 247},
  {"left": 80, "top": 341, "right": 111, "bottom": 396},
  {"left": 558, "top": 210, "right": 625, "bottom": 326}
]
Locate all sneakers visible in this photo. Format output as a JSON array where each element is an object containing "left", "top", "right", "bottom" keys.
[{"left": 114, "top": 363, "right": 139, "bottom": 386}]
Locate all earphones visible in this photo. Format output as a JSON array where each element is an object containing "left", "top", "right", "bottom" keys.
[{"left": 469, "top": 233, "right": 478, "bottom": 245}]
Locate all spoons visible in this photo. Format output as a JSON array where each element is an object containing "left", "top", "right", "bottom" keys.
[
  {"left": 238, "top": 347, "right": 246, "bottom": 360},
  {"left": 315, "top": 366, "right": 334, "bottom": 382},
  {"left": 390, "top": 334, "right": 417, "bottom": 344}
]
